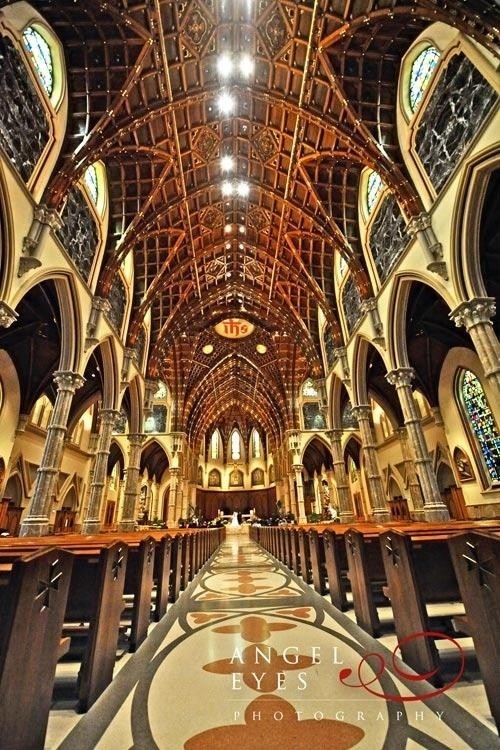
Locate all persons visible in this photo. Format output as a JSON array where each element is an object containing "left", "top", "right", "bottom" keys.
[
  {"left": 231, "top": 510, "right": 242, "bottom": 525},
  {"left": 179, "top": 520, "right": 224, "bottom": 528},
  {"left": 251, "top": 511, "right": 298, "bottom": 526}
]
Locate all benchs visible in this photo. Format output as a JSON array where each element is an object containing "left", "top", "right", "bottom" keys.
[
  {"left": 0, "top": 525, "right": 227, "bottom": 750},
  {"left": 248, "top": 516, "right": 499, "bottom": 736}
]
